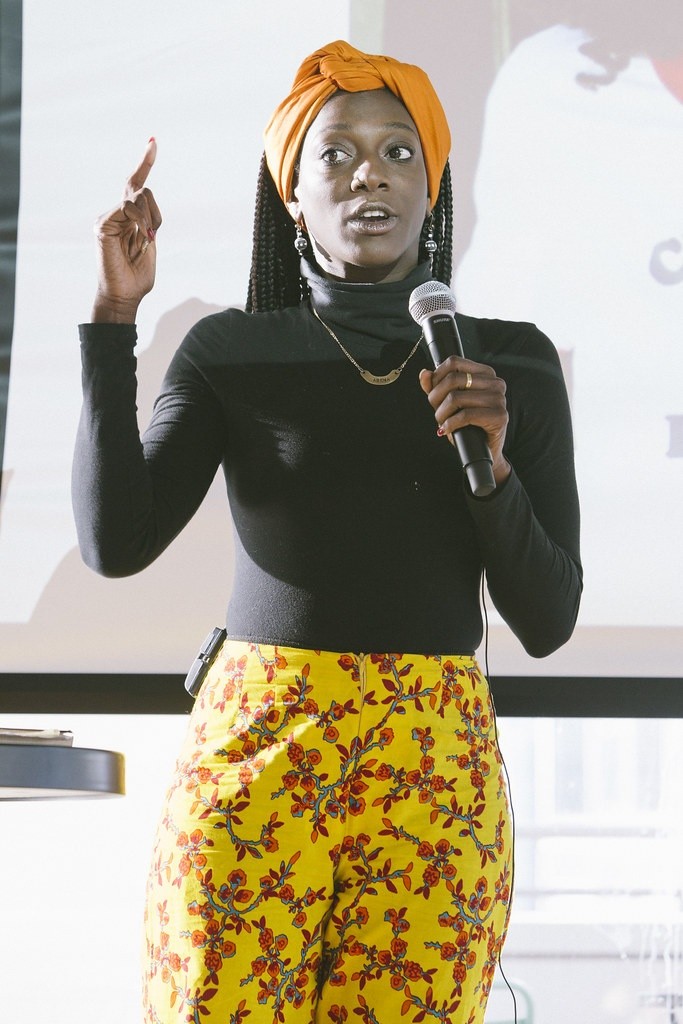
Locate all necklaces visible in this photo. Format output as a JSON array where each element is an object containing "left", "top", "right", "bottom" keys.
[{"left": 314, "top": 308, "right": 426, "bottom": 386}]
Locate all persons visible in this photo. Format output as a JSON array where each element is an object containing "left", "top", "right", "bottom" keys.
[{"left": 71, "top": 41, "right": 584, "bottom": 1024}]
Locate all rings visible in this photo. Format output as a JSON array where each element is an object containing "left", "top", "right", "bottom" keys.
[{"left": 466, "top": 373, "right": 472, "bottom": 390}]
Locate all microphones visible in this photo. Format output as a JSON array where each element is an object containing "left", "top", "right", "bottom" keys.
[{"left": 407, "top": 280, "right": 498, "bottom": 498}]
